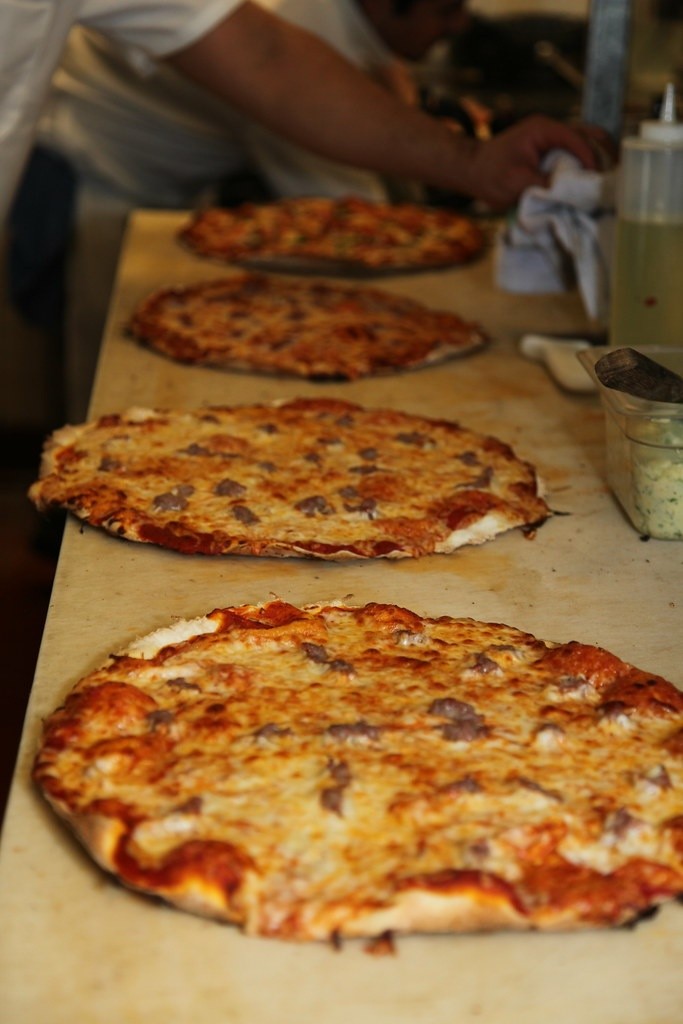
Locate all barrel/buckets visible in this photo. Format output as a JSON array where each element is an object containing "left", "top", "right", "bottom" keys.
[{"left": 574, "top": 345, "right": 683, "bottom": 543}]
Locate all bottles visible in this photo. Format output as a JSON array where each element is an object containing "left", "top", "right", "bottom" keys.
[{"left": 604, "top": 82, "right": 683, "bottom": 351}]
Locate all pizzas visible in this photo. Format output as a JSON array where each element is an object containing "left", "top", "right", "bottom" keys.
[
  {"left": 26, "top": 393, "right": 563, "bottom": 563},
  {"left": 126, "top": 269, "right": 490, "bottom": 381},
  {"left": 33, "top": 590, "right": 683, "bottom": 940},
  {"left": 171, "top": 194, "right": 496, "bottom": 277}
]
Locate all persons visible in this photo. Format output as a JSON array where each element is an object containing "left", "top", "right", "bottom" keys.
[{"left": 0, "top": 0, "right": 620, "bottom": 563}]
